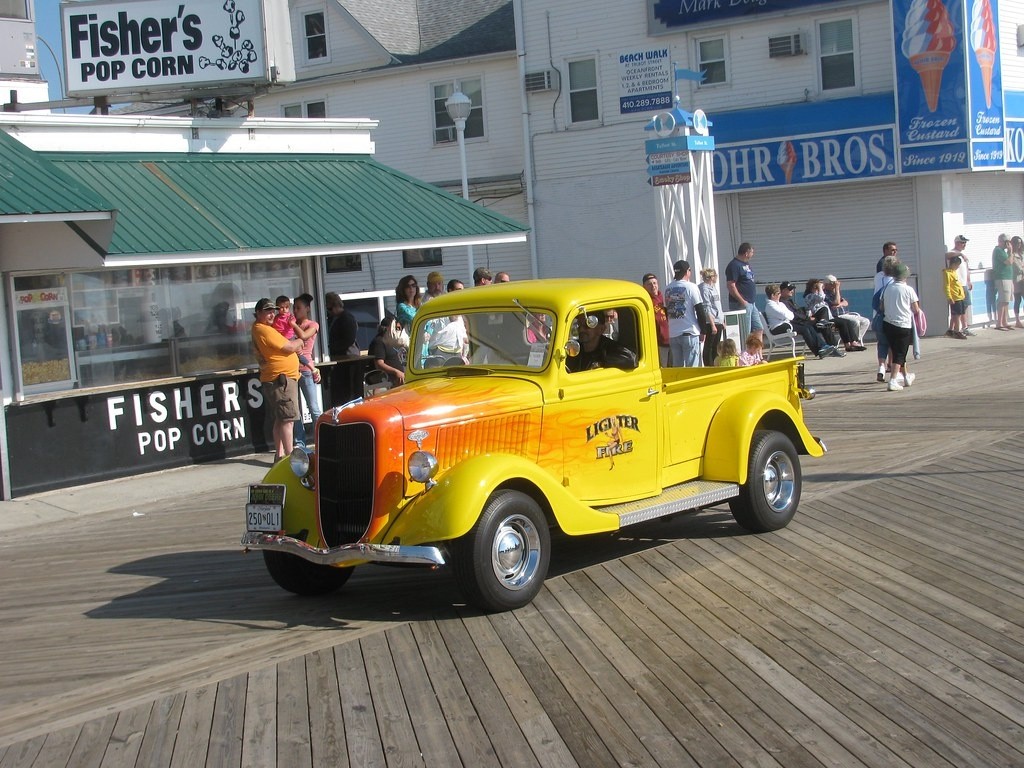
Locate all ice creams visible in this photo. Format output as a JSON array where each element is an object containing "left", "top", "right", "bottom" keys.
[
  {"left": 901, "top": 0, "right": 955, "bottom": 113},
  {"left": 776, "top": 141, "right": 796, "bottom": 184},
  {"left": 970, "top": 0, "right": 997, "bottom": 109}
]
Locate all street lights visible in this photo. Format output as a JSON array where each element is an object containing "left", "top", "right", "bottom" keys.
[{"left": 444, "top": 91, "right": 475, "bottom": 291}]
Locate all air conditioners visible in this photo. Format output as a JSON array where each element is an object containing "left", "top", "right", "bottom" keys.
[
  {"left": 768, "top": 31, "right": 806, "bottom": 58},
  {"left": 525, "top": 68, "right": 559, "bottom": 92}
]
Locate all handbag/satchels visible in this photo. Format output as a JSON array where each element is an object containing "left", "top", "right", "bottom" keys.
[{"left": 872, "top": 311, "right": 884, "bottom": 335}]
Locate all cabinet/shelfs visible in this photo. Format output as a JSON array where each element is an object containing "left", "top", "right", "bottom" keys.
[{"left": 71, "top": 259, "right": 305, "bottom": 329}]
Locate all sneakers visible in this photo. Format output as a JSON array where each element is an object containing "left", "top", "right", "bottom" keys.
[
  {"left": 312, "top": 369, "right": 321, "bottom": 384},
  {"left": 888, "top": 378, "right": 903, "bottom": 391},
  {"left": 896, "top": 372, "right": 904, "bottom": 383},
  {"left": 904, "top": 373, "right": 915, "bottom": 386}
]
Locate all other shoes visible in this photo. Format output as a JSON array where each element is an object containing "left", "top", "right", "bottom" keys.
[
  {"left": 819, "top": 347, "right": 834, "bottom": 359},
  {"left": 1015, "top": 321, "right": 1024, "bottom": 328},
  {"left": 887, "top": 364, "right": 891, "bottom": 371},
  {"left": 877, "top": 366, "right": 886, "bottom": 381},
  {"left": 845, "top": 346, "right": 866, "bottom": 351},
  {"left": 829, "top": 351, "right": 847, "bottom": 357},
  {"left": 963, "top": 328, "right": 977, "bottom": 336},
  {"left": 946, "top": 330, "right": 967, "bottom": 339}
]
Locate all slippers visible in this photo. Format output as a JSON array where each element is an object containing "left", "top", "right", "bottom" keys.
[{"left": 994, "top": 325, "right": 1015, "bottom": 331}]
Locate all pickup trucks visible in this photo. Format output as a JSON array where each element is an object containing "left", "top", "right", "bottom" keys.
[{"left": 240, "top": 273, "right": 830, "bottom": 615}]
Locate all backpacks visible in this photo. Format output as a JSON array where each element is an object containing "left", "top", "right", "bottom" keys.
[{"left": 872, "top": 277, "right": 893, "bottom": 311}]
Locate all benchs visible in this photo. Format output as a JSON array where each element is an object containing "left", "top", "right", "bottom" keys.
[{"left": 757, "top": 303, "right": 862, "bottom": 362}]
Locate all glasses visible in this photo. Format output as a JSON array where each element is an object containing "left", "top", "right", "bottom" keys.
[
  {"left": 501, "top": 280, "right": 505, "bottom": 283},
  {"left": 404, "top": 283, "right": 417, "bottom": 288},
  {"left": 604, "top": 315, "right": 614, "bottom": 319},
  {"left": 327, "top": 308, "right": 333, "bottom": 311},
  {"left": 891, "top": 249, "right": 897, "bottom": 251}
]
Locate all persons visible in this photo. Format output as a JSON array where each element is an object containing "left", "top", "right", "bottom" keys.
[
  {"left": 739, "top": 332, "right": 768, "bottom": 366},
  {"left": 395, "top": 275, "right": 434, "bottom": 369},
  {"left": 600, "top": 308, "right": 619, "bottom": 341},
  {"left": 325, "top": 292, "right": 360, "bottom": 361},
  {"left": 494, "top": 271, "right": 510, "bottom": 283},
  {"left": 368, "top": 314, "right": 405, "bottom": 389},
  {"left": 804, "top": 275, "right": 871, "bottom": 352},
  {"left": 665, "top": 260, "right": 707, "bottom": 367},
  {"left": 643, "top": 273, "right": 673, "bottom": 368},
  {"left": 942, "top": 235, "right": 978, "bottom": 339},
  {"left": 471, "top": 267, "right": 506, "bottom": 365},
  {"left": 871, "top": 242, "right": 920, "bottom": 391},
  {"left": 764, "top": 281, "right": 847, "bottom": 359},
  {"left": 725, "top": 243, "right": 764, "bottom": 352},
  {"left": 697, "top": 268, "right": 726, "bottom": 367},
  {"left": 287, "top": 293, "right": 322, "bottom": 448},
  {"left": 714, "top": 340, "right": 739, "bottom": 367},
  {"left": 271, "top": 296, "right": 321, "bottom": 384},
  {"left": 252, "top": 299, "right": 305, "bottom": 464},
  {"left": 992, "top": 233, "right": 1024, "bottom": 331},
  {"left": 421, "top": 271, "right": 463, "bottom": 364},
  {"left": 567, "top": 310, "right": 639, "bottom": 369},
  {"left": 447, "top": 279, "right": 470, "bottom": 365},
  {"left": 527, "top": 313, "right": 548, "bottom": 344}
]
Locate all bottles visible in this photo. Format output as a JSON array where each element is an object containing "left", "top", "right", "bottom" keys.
[{"left": 76, "top": 332, "right": 113, "bottom": 351}]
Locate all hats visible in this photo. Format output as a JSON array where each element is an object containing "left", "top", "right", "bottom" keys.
[
  {"left": 427, "top": 271, "right": 444, "bottom": 284},
  {"left": 674, "top": 260, "right": 690, "bottom": 279},
  {"left": 255, "top": 298, "right": 280, "bottom": 309},
  {"left": 955, "top": 235, "right": 969, "bottom": 243},
  {"left": 473, "top": 267, "right": 496, "bottom": 280},
  {"left": 643, "top": 274, "right": 657, "bottom": 283},
  {"left": 780, "top": 281, "right": 796, "bottom": 290}
]
